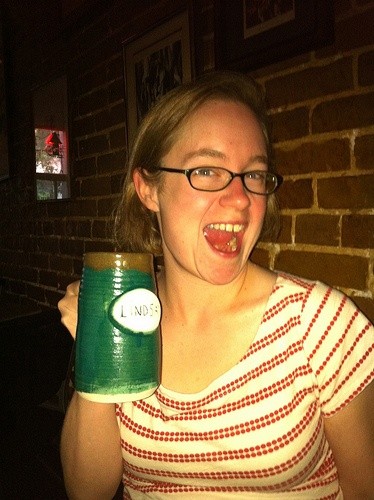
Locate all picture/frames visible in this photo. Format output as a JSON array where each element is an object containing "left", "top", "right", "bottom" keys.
[
  {"left": 120, "top": 5, "right": 200, "bottom": 163},
  {"left": 213, "top": 0, "right": 335, "bottom": 73}
]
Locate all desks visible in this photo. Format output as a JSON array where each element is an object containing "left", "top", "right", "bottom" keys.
[{"left": 0, "top": 300, "right": 44, "bottom": 323}]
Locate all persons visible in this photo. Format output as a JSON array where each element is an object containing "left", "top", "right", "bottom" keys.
[{"left": 54, "top": 73, "right": 373, "bottom": 500}]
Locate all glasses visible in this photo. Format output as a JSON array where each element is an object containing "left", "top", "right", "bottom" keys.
[{"left": 153, "top": 166, "right": 284, "bottom": 195}]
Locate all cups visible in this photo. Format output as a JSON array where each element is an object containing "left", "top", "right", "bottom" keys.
[{"left": 69, "top": 250, "right": 163, "bottom": 403}]
